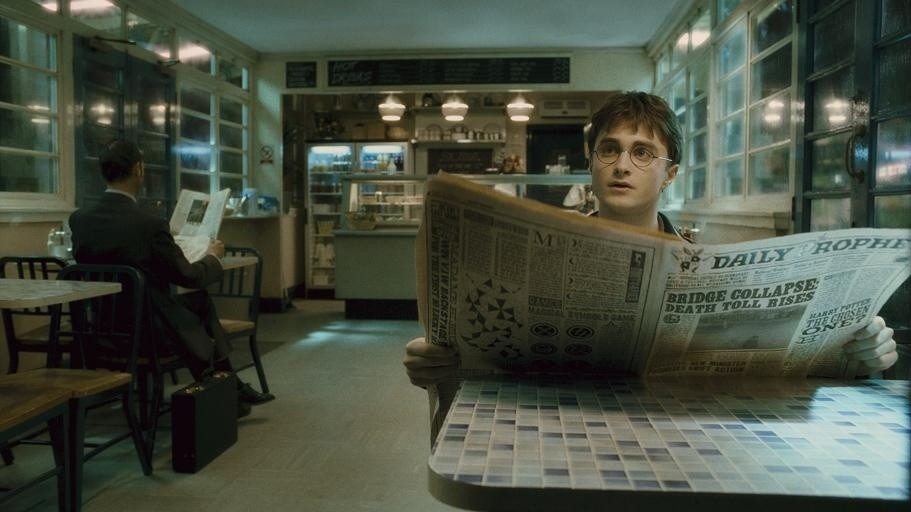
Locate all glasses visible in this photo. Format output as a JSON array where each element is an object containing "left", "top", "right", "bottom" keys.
[{"left": 591, "top": 140, "right": 673, "bottom": 167}]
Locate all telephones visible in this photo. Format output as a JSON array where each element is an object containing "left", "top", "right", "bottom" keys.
[{"left": 503, "top": 155, "right": 522, "bottom": 173}]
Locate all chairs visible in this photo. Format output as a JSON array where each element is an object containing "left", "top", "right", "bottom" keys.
[
  {"left": 1, "top": 255, "right": 96, "bottom": 435},
  {"left": 4, "top": 378, "right": 74, "bottom": 509},
  {"left": 2, "top": 262, "right": 154, "bottom": 510},
  {"left": 76, "top": 252, "right": 194, "bottom": 428},
  {"left": 209, "top": 243, "right": 272, "bottom": 403}
]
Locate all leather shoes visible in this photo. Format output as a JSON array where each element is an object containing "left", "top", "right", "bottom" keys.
[{"left": 238, "top": 381, "right": 276, "bottom": 419}]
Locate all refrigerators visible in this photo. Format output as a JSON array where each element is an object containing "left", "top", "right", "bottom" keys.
[{"left": 303, "top": 139, "right": 414, "bottom": 296}]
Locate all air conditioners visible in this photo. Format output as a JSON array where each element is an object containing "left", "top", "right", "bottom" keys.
[{"left": 539, "top": 99, "right": 591, "bottom": 119}]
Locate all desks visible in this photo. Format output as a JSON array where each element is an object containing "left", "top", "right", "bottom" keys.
[
  {"left": 3, "top": 278, "right": 122, "bottom": 369},
  {"left": 175, "top": 254, "right": 259, "bottom": 298}
]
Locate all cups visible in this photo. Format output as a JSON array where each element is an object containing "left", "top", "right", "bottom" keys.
[
  {"left": 545, "top": 153, "right": 571, "bottom": 175},
  {"left": 48, "top": 230, "right": 70, "bottom": 266}
]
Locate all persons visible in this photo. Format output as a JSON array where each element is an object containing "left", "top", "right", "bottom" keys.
[
  {"left": 495, "top": 155, "right": 527, "bottom": 199},
  {"left": 402, "top": 92, "right": 900, "bottom": 390},
  {"left": 68, "top": 139, "right": 277, "bottom": 404}
]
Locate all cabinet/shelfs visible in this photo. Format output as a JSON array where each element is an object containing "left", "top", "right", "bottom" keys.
[{"left": 334, "top": 171, "right": 591, "bottom": 319}]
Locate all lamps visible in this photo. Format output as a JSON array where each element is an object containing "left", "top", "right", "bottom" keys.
[
  {"left": 502, "top": 93, "right": 533, "bottom": 123},
  {"left": 440, "top": 91, "right": 469, "bottom": 121},
  {"left": 377, "top": 94, "right": 406, "bottom": 121}
]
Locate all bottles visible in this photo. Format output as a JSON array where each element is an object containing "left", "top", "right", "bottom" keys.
[
  {"left": 290, "top": 182, "right": 300, "bottom": 216},
  {"left": 364, "top": 153, "right": 404, "bottom": 171}
]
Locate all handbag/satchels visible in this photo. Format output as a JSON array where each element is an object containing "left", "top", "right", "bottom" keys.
[{"left": 172, "top": 369, "right": 238, "bottom": 474}]
